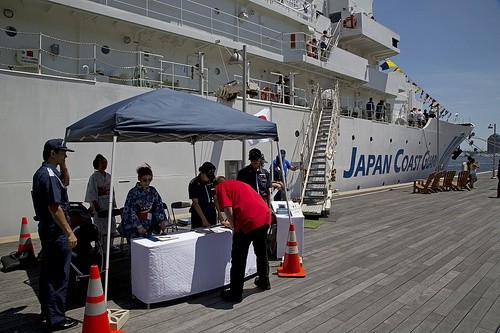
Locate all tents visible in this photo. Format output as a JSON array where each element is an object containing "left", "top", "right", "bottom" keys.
[{"left": 64, "top": 87, "right": 291, "bottom": 308}]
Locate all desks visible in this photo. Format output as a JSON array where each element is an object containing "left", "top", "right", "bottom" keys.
[
  {"left": 272, "top": 200, "right": 304, "bottom": 258},
  {"left": 130, "top": 225, "right": 256, "bottom": 303}
]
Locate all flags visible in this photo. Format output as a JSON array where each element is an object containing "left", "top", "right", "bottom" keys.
[{"left": 245, "top": 108, "right": 272, "bottom": 147}]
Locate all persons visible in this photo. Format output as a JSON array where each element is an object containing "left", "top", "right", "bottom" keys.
[
  {"left": 465, "top": 155, "right": 480, "bottom": 190},
  {"left": 305, "top": 30, "right": 333, "bottom": 57},
  {"left": 117, "top": 166, "right": 167, "bottom": 269},
  {"left": 366, "top": 98, "right": 386, "bottom": 121},
  {"left": 237, "top": 148, "right": 283, "bottom": 209},
  {"left": 85, "top": 154, "right": 117, "bottom": 251},
  {"left": 33, "top": 139, "right": 78, "bottom": 333},
  {"left": 408, "top": 108, "right": 436, "bottom": 127},
  {"left": 189, "top": 162, "right": 224, "bottom": 232},
  {"left": 497, "top": 159, "right": 500, "bottom": 198},
  {"left": 275, "top": 76, "right": 289, "bottom": 104},
  {"left": 269, "top": 149, "right": 299, "bottom": 201},
  {"left": 212, "top": 177, "right": 272, "bottom": 304}
]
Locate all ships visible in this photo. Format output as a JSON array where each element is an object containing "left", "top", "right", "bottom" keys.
[{"left": 0, "top": 0, "right": 477, "bottom": 244}]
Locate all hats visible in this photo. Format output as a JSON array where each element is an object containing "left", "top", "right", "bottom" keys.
[
  {"left": 280, "top": 150, "right": 285, "bottom": 156},
  {"left": 44, "top": 139, "right": 75, "bottom": 152},
  {"left": 249, "top": 148, "right": 261, "bottom": 160},
  {"left": 202, "top": 162, "right": 216, "bottom": 179}
]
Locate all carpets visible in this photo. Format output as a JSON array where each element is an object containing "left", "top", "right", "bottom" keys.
[{"left": 304, "top": 220, "right": 325, "bottom": 228}]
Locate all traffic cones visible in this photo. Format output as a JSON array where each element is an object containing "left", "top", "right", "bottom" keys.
[
  {"left": 276, "top": 223, "right": 306, "bottom": 278},
  {"left": 81, "top": 265, "right": 123, "bottom": 333},
  {"left": 17, "top": 217, "right": 35, "bottom": 262}
]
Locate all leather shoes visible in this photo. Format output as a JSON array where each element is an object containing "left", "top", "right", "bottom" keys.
[{"left": 45, "top": 318, "right": 78, "bottom": 332}]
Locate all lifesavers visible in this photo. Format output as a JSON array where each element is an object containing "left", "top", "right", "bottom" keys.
[
  {"left": 305, "top": 39, "right": 318, "bottom": 57},
  {"left": 261, "top": 86, "right": 275, "bottom": 101},
  {"left": 343, "top": 16, "right": 357, "bottom": 29}
]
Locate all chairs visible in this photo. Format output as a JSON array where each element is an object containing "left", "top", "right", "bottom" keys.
[
  {"left": 411, "top": 170, "right": 471, "bottom": 195},
  {"left": 171, "top": 202, "right": 191, "bottom": 231},
  {"left": 99, "top": 209, "right": 129, "bottom": 261},
  {"left": 162, "top": 202, "right": 175, "bottom": 233}
]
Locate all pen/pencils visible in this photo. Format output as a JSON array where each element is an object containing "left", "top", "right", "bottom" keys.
[{"left": 225, "top": 218, "right": 227, "bottom": 222}]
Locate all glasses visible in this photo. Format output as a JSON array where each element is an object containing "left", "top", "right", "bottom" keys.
[{"left": 143, "top": 178, "right": 152, "bottom": 182}]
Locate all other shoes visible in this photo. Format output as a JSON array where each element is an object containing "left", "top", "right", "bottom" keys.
[
  {"left": 220, "top": 289, "right": 243, "bottom": 303},
  {"left": 255, "top": 277, "right": 271, "bottom": 289}
]
